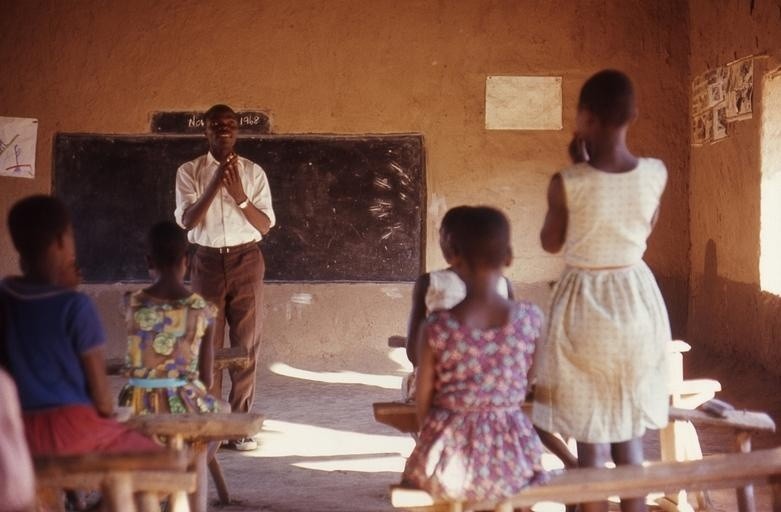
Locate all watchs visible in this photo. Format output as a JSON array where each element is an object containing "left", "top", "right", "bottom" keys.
[{"left": 236, "top": 197, "right": 249, "bottom": 209}]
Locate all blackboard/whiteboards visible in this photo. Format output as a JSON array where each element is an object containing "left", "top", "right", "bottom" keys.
[{"left": 50, "top": 131, "right": 427, "bottom": 283}]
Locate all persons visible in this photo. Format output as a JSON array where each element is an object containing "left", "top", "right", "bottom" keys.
[
  {"left": 117, "top": 222, "right": 231, "bottom": 465},
  {"left": 174, "top": 103, "right": 278, "bottom": 452},
  {"left": 1, "top": 195, "right": 162, "bottom": 455},
  {"left": 399, "top": 206, "right": 547, "bottom": 501},
  {"left": 539, "top": 68, "right": 673, "bottom": 511},
  {"left": 0, "top": 370, "right": 65, "bottom": 512},
  {"left": 402, "top": 207, "right": 476, "bottom": 406}
]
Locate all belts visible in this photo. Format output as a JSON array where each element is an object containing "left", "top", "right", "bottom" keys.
[{"left": 198, "top": 239, "right": 255, "bottom": 255}]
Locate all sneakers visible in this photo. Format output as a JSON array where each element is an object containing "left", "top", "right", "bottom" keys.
[{"left": 230, "top": 438, "right": 257, "bottom": 451}]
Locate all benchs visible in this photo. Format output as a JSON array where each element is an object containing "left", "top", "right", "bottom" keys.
[
  {"left": 388, "top": 447, "right": 781, "bottom": 511},
  {"left": 104, "top": 347, "right": 250, "bottom": 402},
  {"left": 121, "top": 412, "right": 262, "bottom": 510},
  {"left": 388, "top": 335, "right": 693, "bottom": 379},
  {"left": 372, "top": 378, "right": 776, "bottom": 453},
  {"left": 33, "top": 452, "right": 200, "bottom": 512}
]
